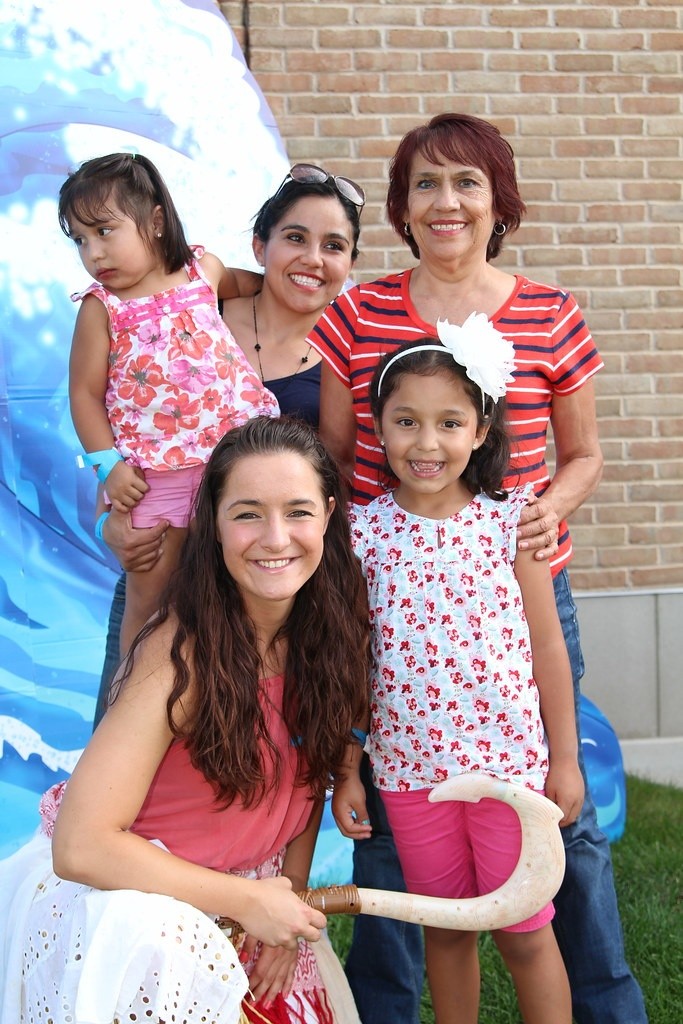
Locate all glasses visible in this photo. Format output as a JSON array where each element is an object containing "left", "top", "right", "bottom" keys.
[{"left": 262, "top": 164, "right": 366, "bottom": 221}]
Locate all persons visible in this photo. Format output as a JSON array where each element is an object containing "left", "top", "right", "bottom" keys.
[{"left": 0, "top": 111, "right": 646, "bottom": 1024}]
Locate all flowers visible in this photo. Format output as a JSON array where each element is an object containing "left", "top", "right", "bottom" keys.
[{"left": 437, "top": 311, "right": 519, "bottom": 405}]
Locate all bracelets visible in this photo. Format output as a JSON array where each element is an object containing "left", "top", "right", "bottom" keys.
[
  {"left": 76, "top": 446, "right": 125, "bottom": 484},
  {"left": 94, "top": 512, "right": 110, "bottom": 539},
  {"left": 345, "top": 728, "right": 366, "bottom": 747}
]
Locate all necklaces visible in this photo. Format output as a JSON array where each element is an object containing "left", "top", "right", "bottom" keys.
[
  {"left": 256, "top": 674, "right": 305, "bottom": 746},
  {"left": 253, "top": 294, "right": 312, "bottom": 396}
]
